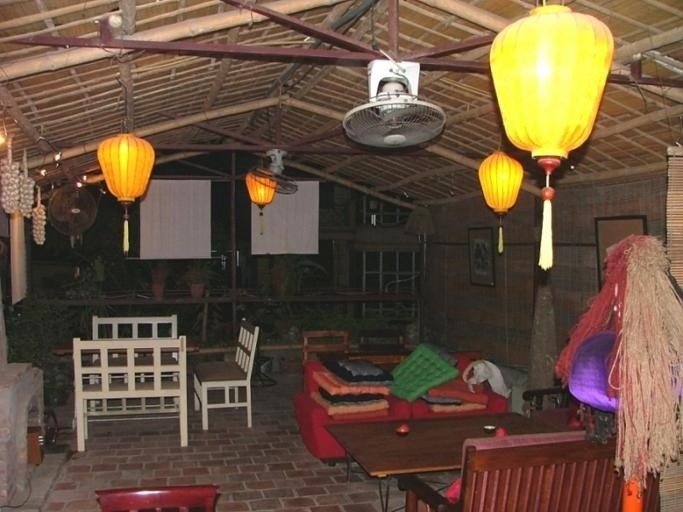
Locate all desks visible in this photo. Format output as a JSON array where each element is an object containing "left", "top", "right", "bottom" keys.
[{"left": 324, "top": 411, "right": 564, "bottom": 512}]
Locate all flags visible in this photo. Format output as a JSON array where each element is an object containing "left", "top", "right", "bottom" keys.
[
  {"left": 249, "top": 181, "right": 320, "bottom": 256},
  {"left": 138, "top": 180, "right": 211, "bottom": 260}
]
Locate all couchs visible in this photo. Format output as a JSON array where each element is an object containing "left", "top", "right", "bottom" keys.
[{"left": 296, "top": 352, "right": 508, "bottom": 467}]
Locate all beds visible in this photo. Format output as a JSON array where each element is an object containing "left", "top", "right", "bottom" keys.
[{"left": 74, "top": 315, "right": 195, "bottom": 450}]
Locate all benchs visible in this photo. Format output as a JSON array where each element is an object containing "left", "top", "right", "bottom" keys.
[{"left": 406, "top": 431, "right": 663, "bottom": 512}]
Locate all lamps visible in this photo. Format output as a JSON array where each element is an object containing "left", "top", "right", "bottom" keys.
[
  {"left": 244, "top": 170, "right": 277, "bottom": 234},
  {"left": 477, "top": 1, "right": 614, "bottom": 270}
]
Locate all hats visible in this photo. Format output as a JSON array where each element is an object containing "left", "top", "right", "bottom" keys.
[{"left": 568, "top": 332, "right": 682, "bottom": 412}]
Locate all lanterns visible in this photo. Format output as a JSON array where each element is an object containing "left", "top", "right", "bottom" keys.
[
  {"left": 242, "top": 169, "right": 278, "bottom": 240},
  {"left": 475, "top": 149, "right": 523, "bottom": 257},
  {"left": 485, "top": 3, "right": 615, "bottom": 275}
]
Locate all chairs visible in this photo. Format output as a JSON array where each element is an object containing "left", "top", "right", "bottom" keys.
[
  {"left": 523, "top": 386, "right": 587, "bottom": 430},
  {"left": 97, "top": 483, "right": 220, "bottom": 512},
  {"left": 193, "top": 317, "right": 261, "bottom": 431},
  {"left": 302, "top": 330, "right": 411, "bottom": 366}
]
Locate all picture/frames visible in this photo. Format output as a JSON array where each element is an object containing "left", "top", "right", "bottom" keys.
[
  {"left": 596, "top": 214, "right": 648, "bottom": 292},
  {"left": 465, "top": 226, "right": 496, "bottom": 286}
]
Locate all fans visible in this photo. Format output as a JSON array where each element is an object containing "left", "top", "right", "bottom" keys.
[
  {"left": 249, "top": 149, "right": 298, "bottom": 196},
  {"left": 342, "top": 60, "right": 446, "bottom": 151},
  {"left": 48, "top": 182, "right": 97, "bottom": 248}
]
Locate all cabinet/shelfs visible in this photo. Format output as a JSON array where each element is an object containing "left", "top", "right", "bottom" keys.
[{"left": 44, "top": 291, "right": 419, "bottom": 355}]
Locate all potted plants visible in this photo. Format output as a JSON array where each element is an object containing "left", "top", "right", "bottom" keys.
[{"left": 152, "top": 260, "right": 212, "bottom": 298}]
